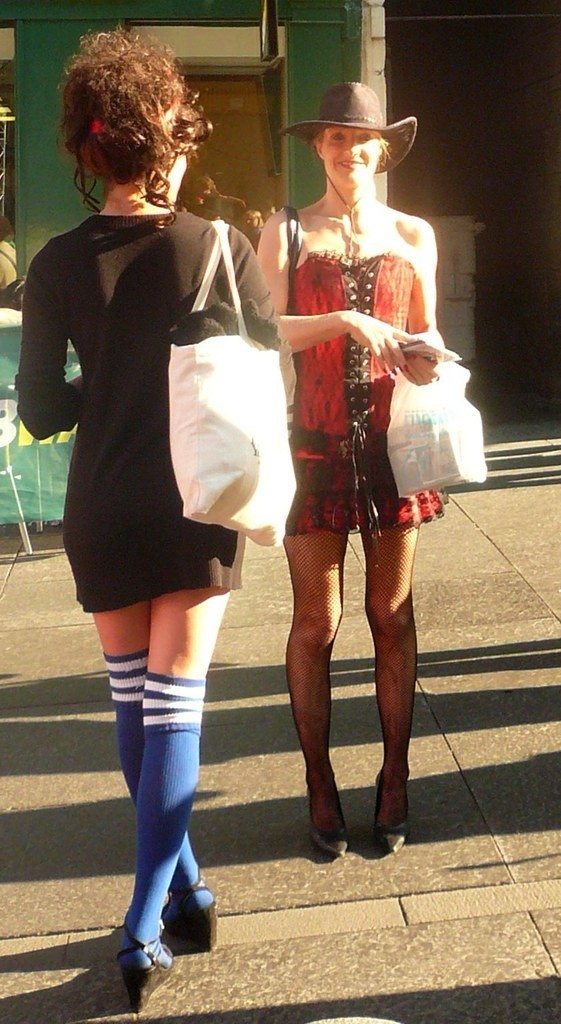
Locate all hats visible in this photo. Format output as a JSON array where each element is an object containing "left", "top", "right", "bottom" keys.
[{"left": 281, "top": 83, "right": 417, "bottom": 173}]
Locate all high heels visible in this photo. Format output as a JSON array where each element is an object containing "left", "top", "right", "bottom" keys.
[
  {"left": 374, "top": 771, "right": 408, "bottom": 853},
  {"left": 117, "top": 911, "right": 173, "bottom": 1014},
  {"left": 308, "top": 773, "right": 347, "bottom": 857},
  {"left": 161, "top": 878, "right": 218, "bottom": 951}
]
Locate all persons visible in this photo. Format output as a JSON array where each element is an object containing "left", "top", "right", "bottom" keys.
[
  {"left": 16, "top": 31, "right": 284, "bottom": 1012},
  {"left": 0, "top": 216, "right": 16, "bottom": 309},
  {"left": 258, "top": 82, "right": 450, "bottom": 857}
]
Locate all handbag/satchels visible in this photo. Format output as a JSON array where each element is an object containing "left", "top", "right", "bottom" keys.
[
  {"left": 386, "top": 349, "right": 488, "bottom": 497},
  {"left": 278, "top": 206, "right": 302, "bottom": 440},
  {"left": 168, "top": 221, "right": 296, "bottom": 546}
]
